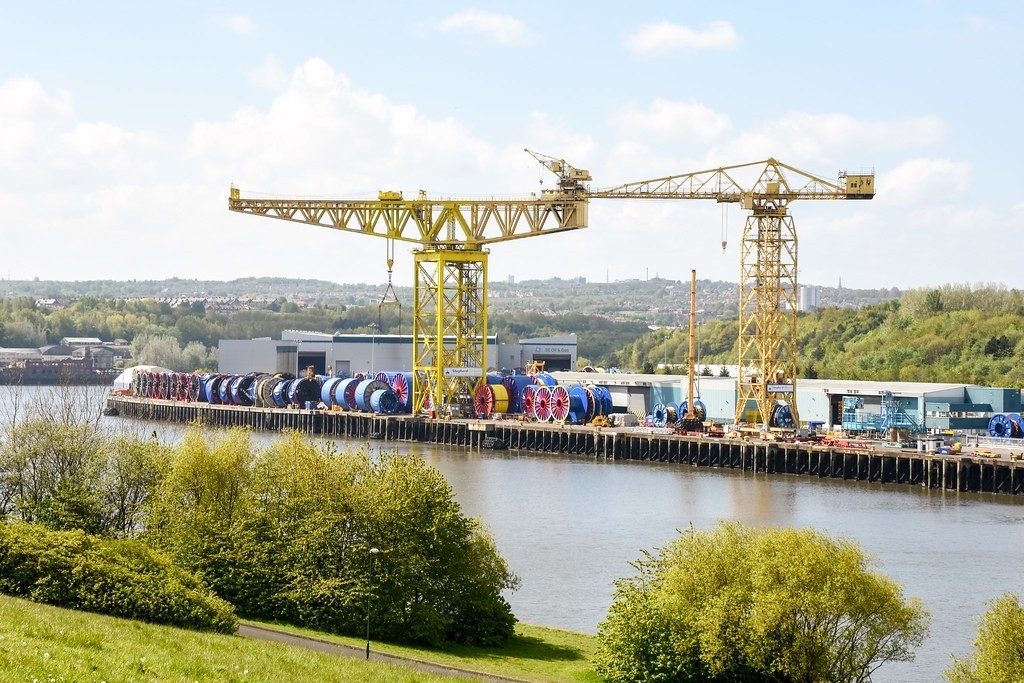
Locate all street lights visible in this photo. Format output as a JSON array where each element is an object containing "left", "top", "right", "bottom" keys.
[{"left": 366, "top": 549, "right": 381, "bottom": 659}]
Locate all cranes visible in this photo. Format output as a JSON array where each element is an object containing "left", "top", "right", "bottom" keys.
[
  {"left": 521, "top": 148, "right": 594, "bottom": 201},
  {"left": 226, "top": 174, "right": 585, "bottom": 412},
  {"left": 577, "top": 151, "right": 878, "bottom": 436}
]
